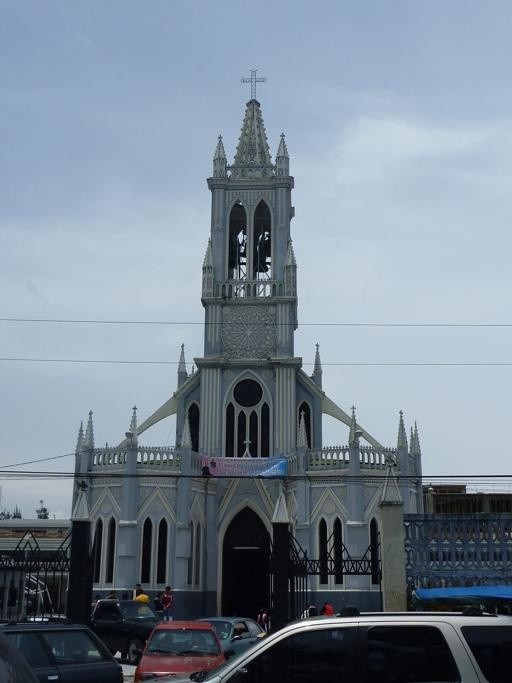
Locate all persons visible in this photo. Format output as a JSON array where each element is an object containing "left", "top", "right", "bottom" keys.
[
  {"left": 154, "top": 591, "right": 163, "bottom": 611},
  {"left": 135, "top": 588, "right": 152, "bottom": 615},
  {"left": 107, "top": 590, "right": 117, "bottom": 599},
  {"left": 257, "top": 601, "right": 317, "bottom": 633},
  {"left": 131, "top": 583, "right": 143, "bottom": 599},
  {"left": 91, "top": 594, "right": 102, "bottom": 609},
  {"left": 160, "top": 585, "right": 174, "bottom": 622}
]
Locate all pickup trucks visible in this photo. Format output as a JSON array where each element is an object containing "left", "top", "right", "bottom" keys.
[{"left": 47, "top": 598, "right": 158, "bottom": 664}]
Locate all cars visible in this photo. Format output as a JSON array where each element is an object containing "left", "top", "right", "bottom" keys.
[
  {"left": 196, "top": 616, "right": 267, "bottom": 665},
  {"left": 0, "top": 619, "right": 123, "bottom": 682},
  {"left": 140, "top": 605, "right": 512, "bottom": 682},
  {"left": 133, "top": 621, "right": 225, "bottom": 682}
]
